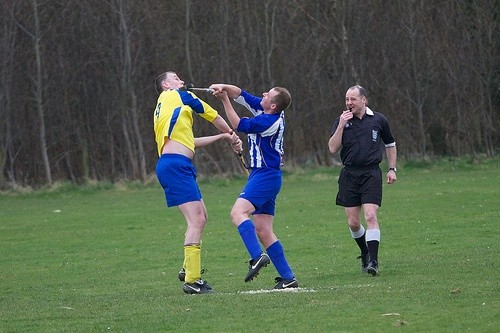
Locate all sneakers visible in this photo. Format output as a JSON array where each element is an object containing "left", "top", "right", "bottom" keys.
[
  {"left": 182, "top": 277, "right": 217, "bottom": 295},
  {"left": 273, "top": 277, "right": 298, "bottom": 289},
  {"left": 366, "top": 260, "right": 378, "bottom": 277},
  {"left": 361, "top": 254, "right": 370, "bottom": 273},
  {"left": 244, "top": 252, "right": 271, "bottom": 283},
  {"left": 177, "top": 268, "right": 185, "bottom": 281}
]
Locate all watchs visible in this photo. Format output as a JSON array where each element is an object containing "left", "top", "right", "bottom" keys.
[{"left": 390, "top": 168, "right": 396, "bottom": 171}]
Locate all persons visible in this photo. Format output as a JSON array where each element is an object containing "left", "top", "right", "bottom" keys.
[
  {"left": 326, "top": 85, "right": 398, "bottom": 276},
  {"left": 154, "top": 70, "right": 244, "bottom": 294},
  {"left": 208, "top": 83, "right": 299, "bottom": 289}
]
